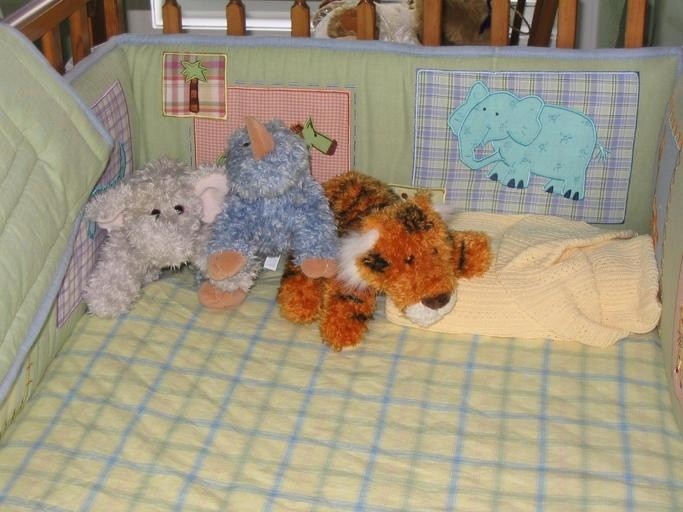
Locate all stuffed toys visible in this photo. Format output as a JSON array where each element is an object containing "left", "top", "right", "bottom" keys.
[
  {"left": 78, "top": 154, "right": 231, "bottom": 321},
  {"left": 275, "top": 168, "right": 489, "bottom": 347},
  {"left": 309, "top": 0, "right": 507, "bottom": 50},
  {"left": 196, "top": 116, "right": 340, "bottom": 312}
]
